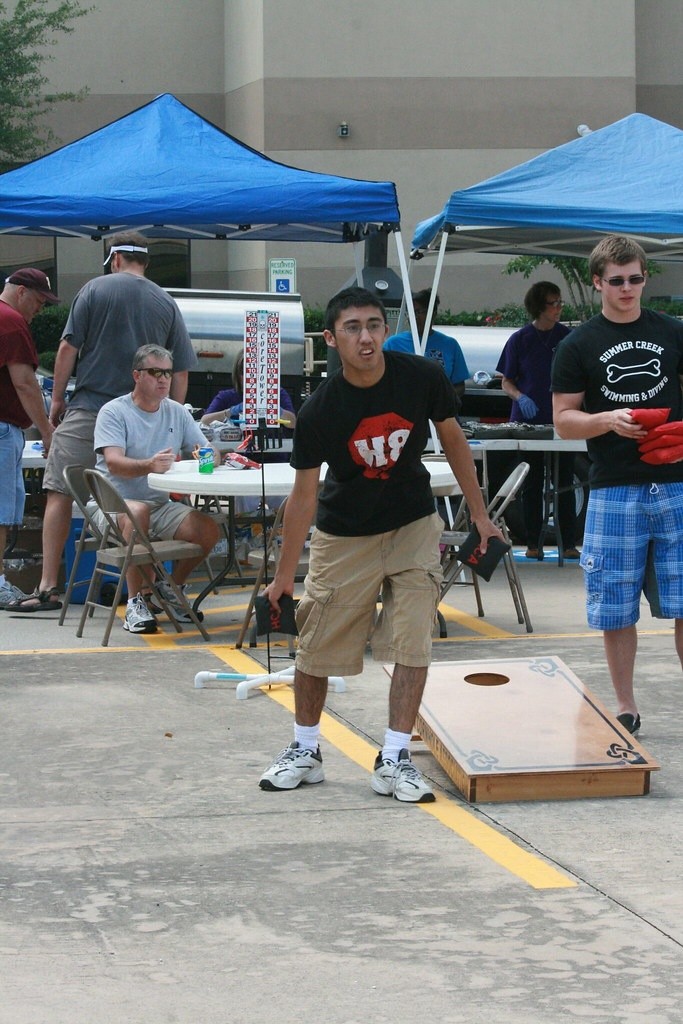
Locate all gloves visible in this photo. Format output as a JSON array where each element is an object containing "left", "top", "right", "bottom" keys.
[
  {"left": 229, "top": 401, "right": 243, "bottom": 415},
  {"left": 518, "top": 395, "right": 539, "bottom": 420},
  {"left": 280, "top": 407, "right": 284, "bottom": 416}
]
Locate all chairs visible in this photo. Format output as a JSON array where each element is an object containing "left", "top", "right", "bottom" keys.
[{"left": 58, "top": 450, "right": 533, "bottom": 649}]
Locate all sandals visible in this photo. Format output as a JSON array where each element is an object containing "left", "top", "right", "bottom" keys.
[
  {"left": 142, "top": 584, "right": 162, "bottom": 614},
  {"left": 30, "top": 584, "right": 62, "bottom": 610},
  {"left": 0, "top": 582, "right": 41, "bottom": 611}
]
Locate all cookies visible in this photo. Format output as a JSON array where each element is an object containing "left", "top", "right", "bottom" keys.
[{"left": 191, "top": 444, "right": 200, "bottom": 459}]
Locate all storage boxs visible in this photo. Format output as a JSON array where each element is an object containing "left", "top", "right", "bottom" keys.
[{"left": 65, "top": 496, "right": 173, "bottom": 606}]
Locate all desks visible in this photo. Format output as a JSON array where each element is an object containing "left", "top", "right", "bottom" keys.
[
  {"left": 147, "top": 459, "right": 463, "bottom": 657},
  {"left": 424, "top": 439, "right": 518, "bottom": 450},
  {"left": 464, "top": 387, "right": 509, "bottom": 396},
  {"left": 518, "top": 439, "right": 590, "bottom": 566},
  {"left": 5, "top": 438, "right": 293, "bottom": 553}
]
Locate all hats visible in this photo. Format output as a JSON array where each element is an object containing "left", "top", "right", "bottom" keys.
[
  {"left": 102, "top": 245, "right": 149, "bottom": 266},
  {"left": 5, "top": 268, "right": 61, "bottom": 304},
  {"left": 413, "top": 287, "right": 440, "bottom": 314}
]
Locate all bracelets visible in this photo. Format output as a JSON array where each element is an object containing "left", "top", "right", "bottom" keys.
[{"left": 224, "top": 409, "right": 227, "bottom": 417}]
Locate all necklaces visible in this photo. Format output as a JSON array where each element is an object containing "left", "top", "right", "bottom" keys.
[{"left": 535, "top": 323, "right": 555, "bottom": 348}]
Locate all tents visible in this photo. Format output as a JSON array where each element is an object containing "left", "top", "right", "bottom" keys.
[
  {"left": 0, "top": 93, "right": 468, "bottom": 585},
  {"left": 395, "top": 113, "right": 683, "bottom": 357}
]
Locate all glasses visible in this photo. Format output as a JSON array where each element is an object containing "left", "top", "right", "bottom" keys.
[
  {"left": 546, "top": 300, "right": 565, "bottom": 308},
  {"left": 330, "top": 324, "right": 386, "bottom": 336},
  {"left": 139, "top": 367, "right": 173, "bottom": 378},
  {"left": 601, "top": 273, "right": 645, "bottom": 286},
  {"left": 404, "top": 309, "right": 426, "bottom": 317}
]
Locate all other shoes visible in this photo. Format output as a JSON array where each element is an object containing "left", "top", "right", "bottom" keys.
[
  {"left": 564, "top": 548, "right": 579, "bottom": 559},
  {"left": 525, "top": 548, "right": 540, "bottom": 558}
]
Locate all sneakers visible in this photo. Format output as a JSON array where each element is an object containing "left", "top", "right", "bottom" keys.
[
  {"left": 122, "top": 592, "right": 157, "bottom": 633},
  {"left": 150, "top": 580, "right": 204, "bottom": 622},
  {"left": 370, "top": 747, "right": 436, "bottom": 803},
  {"left": 615, "top": 712, "right": 641, "bottom": 737},
  {"left": 258, "top": 740, "right": 325, "bottom": 791}
]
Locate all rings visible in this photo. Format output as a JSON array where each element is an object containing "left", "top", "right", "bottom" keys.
[{"left": 49, "top": 415, "right": 55, "bottom": 419}]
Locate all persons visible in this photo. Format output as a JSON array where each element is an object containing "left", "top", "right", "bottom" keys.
[
  {"left": 0, "top": 229, "right": 581, "bottom": 634},
  {"left": 258, "top": 287, "right": 505, "bottom": 803},
  {"left": 536, "top": 237, "right": 683, "bottom": 738}
]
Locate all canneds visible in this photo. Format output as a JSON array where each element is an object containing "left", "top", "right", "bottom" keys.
[{"left": 198, "top": 446, "right": 214, "bottom": 475}]
